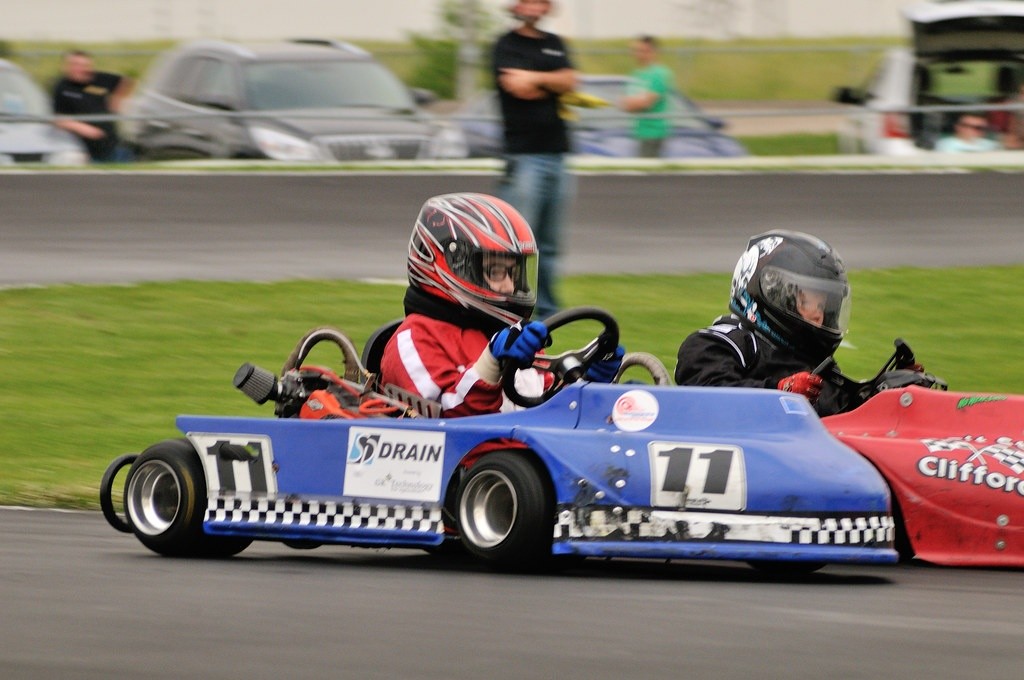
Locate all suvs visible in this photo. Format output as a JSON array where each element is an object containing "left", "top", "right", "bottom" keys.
[
  {"left": 833, "top": 1, "right": 1024, "bottom": 160},
  {"left": 109, "top": 31, "right": 474, "bottom": 164}
]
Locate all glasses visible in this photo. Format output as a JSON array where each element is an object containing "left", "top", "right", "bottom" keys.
[
  {"left": 959, "top": 121, "right": 989, "bottom": 134},
  {"left": 483, "top": 261, "right": 522, "bottom": 281}
]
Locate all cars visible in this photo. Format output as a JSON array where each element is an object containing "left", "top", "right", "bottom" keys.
[
  {"left": 464, "top": 74, "right": 754, "bottom": 160},
  {"left": 0, "top": 55, "right": 93, "bottom": 168}
]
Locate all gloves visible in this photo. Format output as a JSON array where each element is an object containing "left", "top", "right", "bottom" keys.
[
  {"left": 778, "top": 370, "right": 826, "bottom": 406},
  {"left": 907, "top": 363, "right": 925, "bottom": 372},
  {"left": 489, "top": 318, "right": 549, "bottom": 370},
  {"left": 580, "top": 346, "right": 626, "bottom": 382}
]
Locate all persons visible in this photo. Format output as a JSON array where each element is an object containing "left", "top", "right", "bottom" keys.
[
  {"left": 914, "top": 65, "right": 1024, "bottom": 152},
  {"left": 49, "top": 49, "right": 137, "bottom": 162},
  {"left": 381, "top": 192, "right": 625, "bottom": 418},
  {"left": 616, "top": 34, "right": 672, "bottom": 158},
  {"left": 489, "top": 0, "right": 581, "bottom": 318},
  {"left": 674, "top": 229, "right": 926, "bottom": 418}
]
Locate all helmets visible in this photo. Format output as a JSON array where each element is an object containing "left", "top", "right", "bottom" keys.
[
  {"left": 407, "top": 193, "right": 539, "bottom": 331},
  {"left": 729, "top": 230, "right": 849, "bottom": 362}
]
